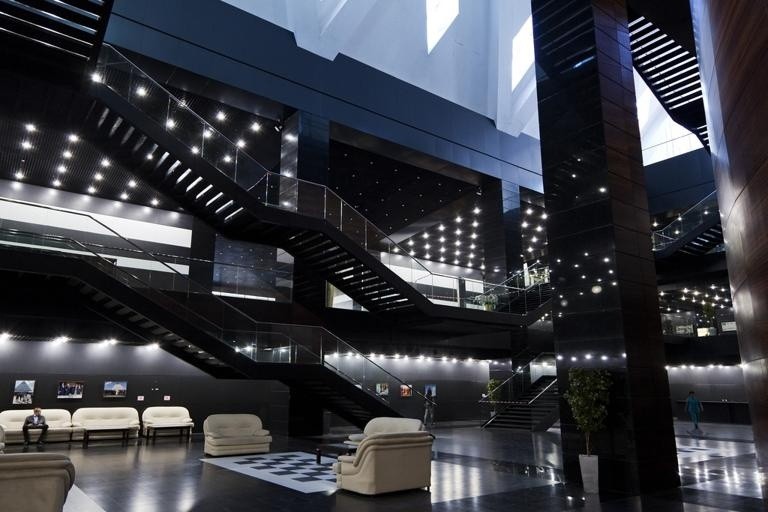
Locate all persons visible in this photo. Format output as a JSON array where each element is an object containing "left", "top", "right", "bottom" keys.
[
  {"left": 22, "top": 407, "right": 49, "bottom": 446},
  {"left": 422, "top": 388, "right": 436, "bottom": 428},
  {"left": 684, "top": 390, "right": 704, "bottom": 431}
]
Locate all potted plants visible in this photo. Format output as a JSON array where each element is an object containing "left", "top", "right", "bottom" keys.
[
  {"left": 486, "top": 378, "right": 506, "bottom": 418},
  {"left": 475, "top": 293, "right": 500, "bottom": 311},
  {"left": 563, "top": 363, "right": 615, "bottom": 494}
]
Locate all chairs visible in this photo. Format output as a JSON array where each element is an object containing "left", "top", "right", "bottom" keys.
[{"left": 0, "top": 452, "right": 76, "bottom": 512}]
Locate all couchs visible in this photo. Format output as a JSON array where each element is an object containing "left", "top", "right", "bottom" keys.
[
  {"left": 331, "top": 415, "right": 436, "bottom": 498},
  {"left": 202, "top": 413, "right": 273, "bottom": 457},
  {"left": 0, "top": 405, "right": 197, "bottom": 445}
]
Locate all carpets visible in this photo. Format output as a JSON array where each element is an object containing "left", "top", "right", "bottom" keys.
[{"left": 199, "top": 449, "right": 342, "bottom": 495}]
